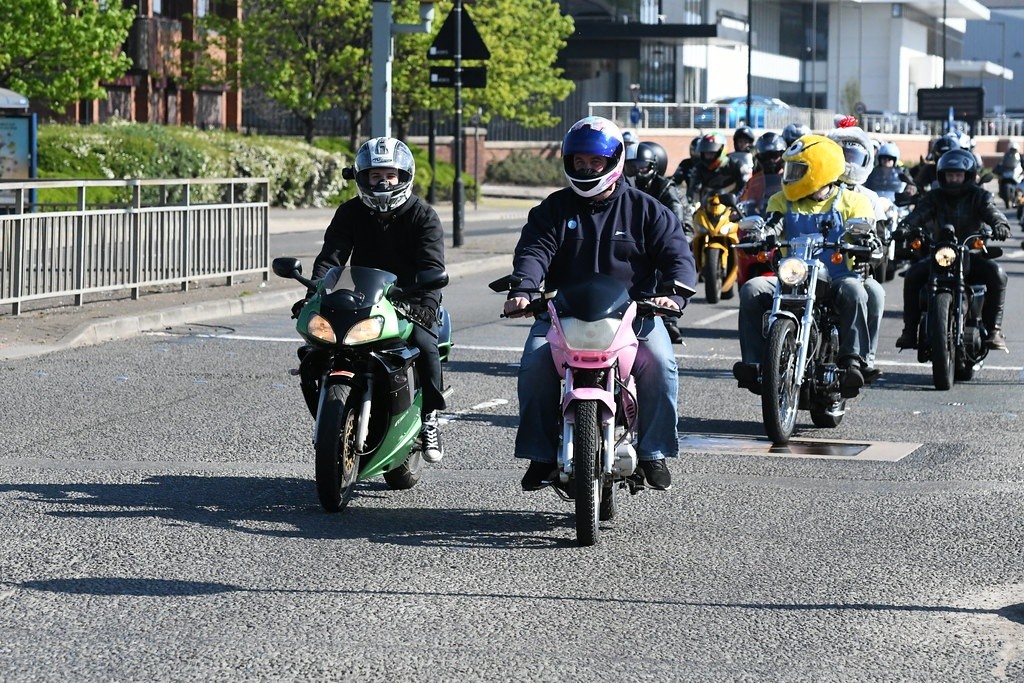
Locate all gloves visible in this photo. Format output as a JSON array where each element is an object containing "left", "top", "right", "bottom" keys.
[
  {"left": 846, "top": 238, "right": 877, "bottom": 259},
  {"left": 412, "top": 303, "right": 436, "bottom": 329},
  {"left": 739, "top": 233, "right": 763, "bottom": 256},
  {"left": 991, "top": 223, "right": 1011, "bottom": 242},
  {"left": 291, "top": 293, "right": 315, "bottom": 319},
  {"left": 891, "top": 221, "right": 911, "bottom": 243}
]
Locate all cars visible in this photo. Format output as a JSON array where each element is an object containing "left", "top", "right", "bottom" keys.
[{"left": 695, "top": 96, "right": 791, "bottom": 128}]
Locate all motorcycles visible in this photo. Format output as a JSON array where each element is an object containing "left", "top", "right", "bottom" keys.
[
  {"left": 488, "top": 273, "right": 698, "bottom": 546},
  {"left": 272, "top": 257, "right": 454, "bottom": 512},
  {"left": 996, "top": 148, "right": 1024, "bottom": 210},
  {"left": 887, "top": 222, "right": 1007, "bottom": 390},
  {"left": 689, "top": 185, "right": 1023, "bottom": 305},
  {"left": 730, "top": 216, "right": 891, "bottom": 444}
]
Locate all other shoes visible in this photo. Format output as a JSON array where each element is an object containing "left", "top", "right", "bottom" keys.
[
  {"left": 860, "top": 364, "right": 883, "bottom": 383},
  {"left": 733, "top": 362, "right": 762, "bottom": 395},
  {"left": 988, "top": 330, "right": 1007, "bottom": 351},
  {"left": 896, "top": 328, "right": 917, "bottom": 348},
  {"left": 838, "top": 366, "right": 864, "bottom": 399}
]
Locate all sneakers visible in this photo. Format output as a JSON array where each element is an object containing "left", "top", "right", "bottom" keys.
[
  {"left": 420, "top": 414, "right": 444, "bottom": 464},
  {"left": 521, "top": 460, "right": 561, "bottom": 492},
  {"left": 636, "top": 458, "right": 672, "bottom": 492}
]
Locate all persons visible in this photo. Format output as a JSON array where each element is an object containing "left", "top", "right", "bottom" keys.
[
  {"left": 291, "top": 136, "right": 446, "bottom": 463},
  {"left": 999, "top": 143, "right": 1023, "bottom": 232},
  {"left": 863, "top": 131, "right": 985, "bottom": 223},
  {"left": 502, "top": 116, "right": 699, "bottom": 490},
  {"left": 623, "top": 123, "right": 814, "bottom": 252},
  {"left": 733, "top": 126, "right": 885, "bottom": 399},
  {"left": 892, "top": 148, "right": 1012, "bottom": 349}
]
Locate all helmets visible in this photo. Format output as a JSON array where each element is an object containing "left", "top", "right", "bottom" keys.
[
  {"left": 1008, "top": 142, "right": 1021, "bottom": 153},
  {"left": 825, "top": 126, "right": 874, "bottom": 186},
  {"left": 733, "top": 123, "right": 812, "bottom": 162},
  {"left": 560, "top": 116, "right": 626, "bottom": 198},
  {"left": 926, "top": 136, "right": 978, "bottom": 198},
  {"left": 871, "top": 137, "right": 900, "bottom": 168},
  {"left": 780, "top": 134, "right": 846, "bottom": 201},
  {"left": 688, "top": 132, "right": 728, "bottom": 172},
  {"left": 353, "top": 136, "right": 415, "bottom": 212},
  {"left": 621, "top": 131, "right": 668, "bottom": 190}
]
